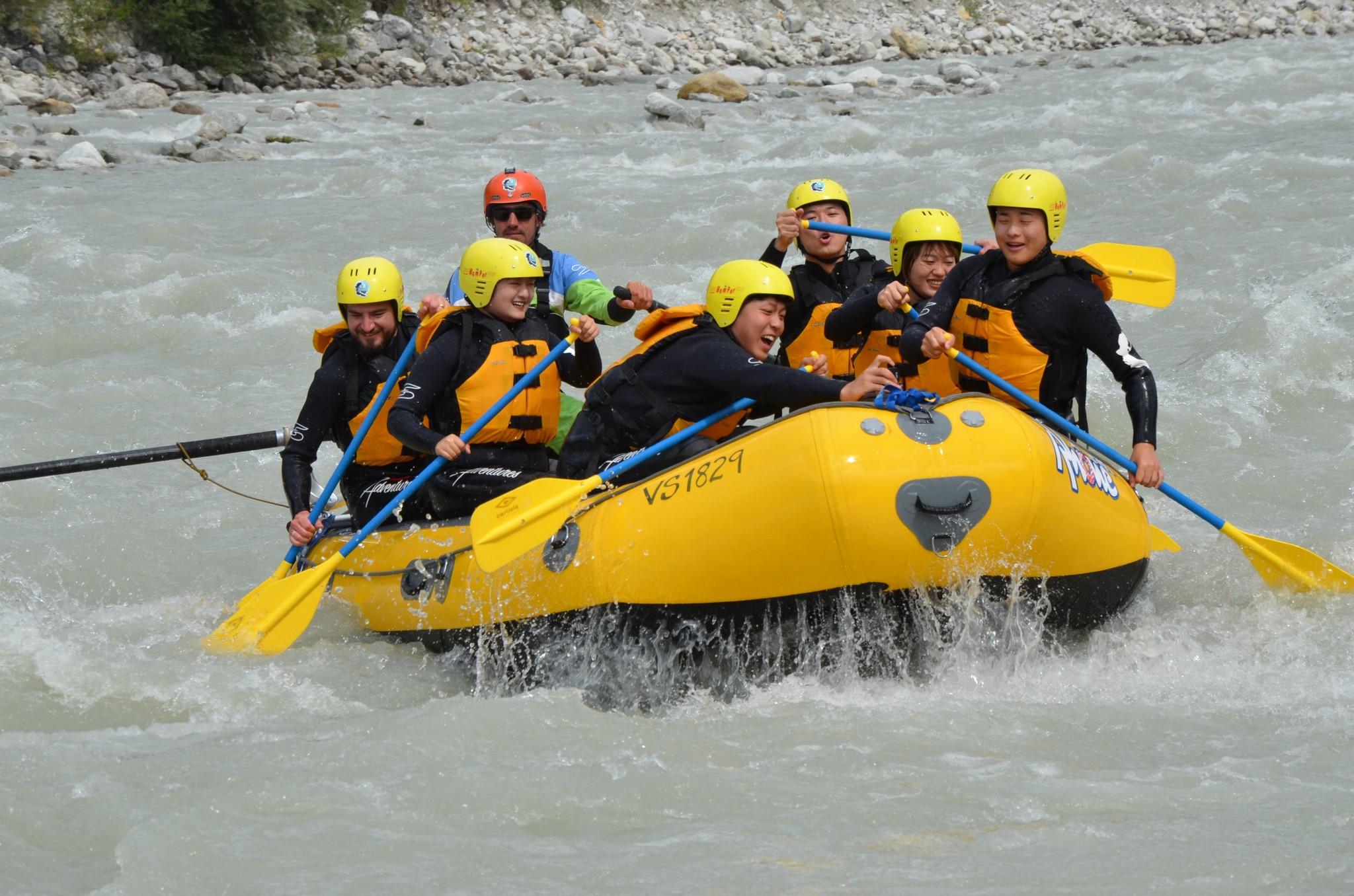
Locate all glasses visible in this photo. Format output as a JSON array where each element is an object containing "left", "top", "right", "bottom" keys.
[{"left": 490, "top": 205, "right": 543, "bottom": 222}]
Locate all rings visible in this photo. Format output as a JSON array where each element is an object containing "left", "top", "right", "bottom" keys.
[
  {"left": 291, "top": 531, "right": 296, "bottom": 536},
  {"left": 439, "top": 304, "right": 445, "bottom": 307}
]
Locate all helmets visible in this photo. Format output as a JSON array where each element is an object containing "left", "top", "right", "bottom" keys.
[
  {"left": 483, "top": 168, "right": 547, "bottom": 219},
  {"left": 890, "top": 208, "right": 964, "bottom": 278},
  {"left": 336, "top": 256, "right": 404, "bottom": 328},
  {"left": 459, "top": 237, "right": 544, "bottom": 310},
  {"left": 786, "top": 178, "right": 853, "bottom": 250},
  {"left": 985, "top": 167, "right": 1067, "bottom": 243},
  {"left": 706, "top": 259, "right": 795, "bottom": 328}
]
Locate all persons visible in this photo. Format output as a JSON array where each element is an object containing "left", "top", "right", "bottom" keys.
[
  {"left": 899, "top": 168, "right": 1164, "bottom": 488},
  {"left": 756, "top": 178, "right": 1000, "bottom": 416},
  {"left": 388, "top": 238, "right": 600, "bottom": 517},
  {"left": 281, "top": 256, "right": 451, "bottom": 546},
  {"left": 555, "top": 260, "right": 902, "bottom": 495},
  {"left": 442, "top": 168, "right": 653, "bottom": 459}
]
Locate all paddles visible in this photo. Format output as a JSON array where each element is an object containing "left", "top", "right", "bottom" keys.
[
  {"left": 469, "top": 351, "right": 821, "bottom": 573},
  {"left": 790, "top": 207, "right": 1176, "bottom": 309},
  {"left": 901, "top": 286, "right": 1182, "bottom": 554},
  {"left": 614, "top": 286, "right": 670, "bottom": 314},
  {"left": 0, "top": 425, "right": 337, "bottom": 483},
  {"left": 218, "top": 301, "right": 429, "bottom": 614},
  {"left": 943, "top": 333, "right": 1354, "bottom": 597},
  {"left": 203, "top": 317, "right": 582, "bottom": 654}
]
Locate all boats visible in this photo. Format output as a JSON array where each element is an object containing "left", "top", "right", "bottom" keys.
[{"left": 292, "top": 390, "right": 1180, "bottom": 718}]
